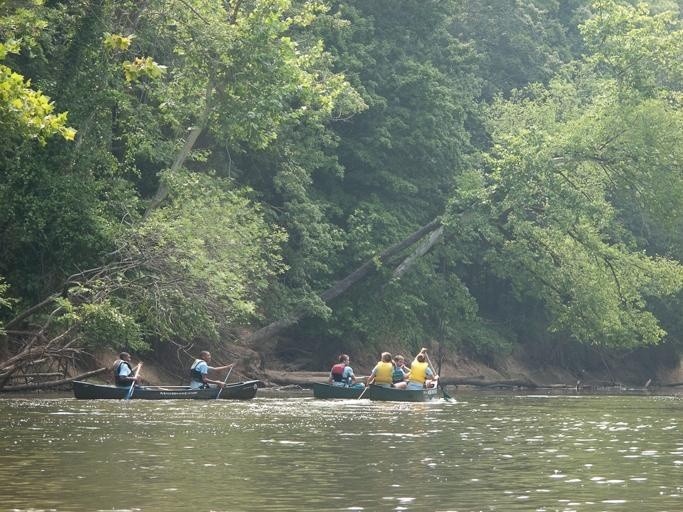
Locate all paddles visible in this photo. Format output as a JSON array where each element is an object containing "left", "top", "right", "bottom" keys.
[
  {"left": 424, "top": 352, "right": 450, "bottom": 401},
  {"left": 124, "top": 363, "right": 141, "bottom": 399}
]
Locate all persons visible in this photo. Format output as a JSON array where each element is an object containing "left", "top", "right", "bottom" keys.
[
  {"left": 110, "top": 350, "right": 147, "bottom": 386},
  {"left": 404, "top": 346, "right": 439, "bottom": 389},
  {"left": 364, "top": 352, "right": 409, "bottom": 389},
  {"left": 390, "top": 354, "right": 410, "bottom": 386},
  {"left": 189, "top": 349, "right": 236, "bottom": 389},
  {"left": 325, "top": 353, "right": 364, "bottom": 389}
]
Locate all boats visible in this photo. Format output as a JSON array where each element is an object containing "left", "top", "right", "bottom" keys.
[
  {"left": 72, "top": 380, "right": 261, "bottom": 399},
  {"left": 312, "top": 380, "right": 438, "bottom": 402}
]
